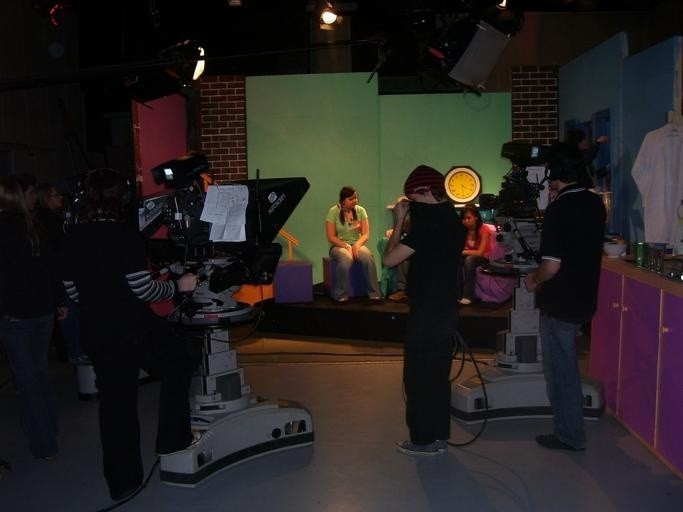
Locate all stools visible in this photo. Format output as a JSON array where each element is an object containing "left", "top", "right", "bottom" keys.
[
  {"left": 274, "top": 260, "right": 313, "bottom": 305},
  {"left": 322, "top": 256, "right": 366, "bottom": 298}
]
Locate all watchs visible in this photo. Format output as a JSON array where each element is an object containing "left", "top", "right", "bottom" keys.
[{"left": 346, "top": 245, "right": 351, "bottom": 248}]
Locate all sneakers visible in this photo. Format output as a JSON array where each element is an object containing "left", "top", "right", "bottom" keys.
[
  {"left": 458, "top": 298, "right": 471, "bottom": 305},
  {"left": 337, "top": 296, "right": 349, "bottom": 302},
  {"left": 389, "top": 291, "right": 408, "bottom": 300},
  {"left": 394, "top": 438, "right": 440, "bottom": 455},
  {"left": 436, "top": 440, "right": 448, "bottom": 453},
  {"left": 368, "top": 293, "right": 381, "bottom": 300},
  {"left": 158, "top": 429, "right": 202, "bottom": 457}
]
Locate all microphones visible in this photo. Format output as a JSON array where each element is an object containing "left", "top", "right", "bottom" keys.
[{"left": 539, "top": 175, "right": 559, "bottom": 190}]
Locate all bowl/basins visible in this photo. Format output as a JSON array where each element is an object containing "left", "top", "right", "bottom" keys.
[{"left": 603, "top": 241, "right": 627, "bottom": 259}]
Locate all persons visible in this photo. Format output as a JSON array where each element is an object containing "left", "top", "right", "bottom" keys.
[
  {"left": 1, "top": 180, "right": 67, "bottom": 458},
  {"left": 325, "top": 186, "right": 384, "bottom": 303},
  {"left": 381, "top": 165, "right": 460, "bottom": 455},
  {"left": 72, "top": 176, "right": 197, "bottom": 499},
  {"left": 39, "top": 184, "right": 65, "bottom": 270},
  {"left": 460, "top": 203, "right": 495, "bottom": 304},
  {"left": 524, "top": 145, "right": 610, "bottom": 453}
]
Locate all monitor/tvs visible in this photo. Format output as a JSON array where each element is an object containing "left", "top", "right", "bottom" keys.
[{"left": 234, "top": 179, "right": 311, "bottom": 242}]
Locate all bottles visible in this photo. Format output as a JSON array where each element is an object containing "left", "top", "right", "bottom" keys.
[
  {"left": 616, "top": 231, "right": 624, "bottom": 247},
  {"left": 634, "top": 242, "right": 646, "bottom": 268}
]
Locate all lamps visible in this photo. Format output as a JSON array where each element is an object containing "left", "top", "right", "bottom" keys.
[
  {"left": 446, "top": 0, "right": 524, "bottom": 90},
  {"left": 317, "top": 0, "right": 342, "bottom": 48},
  {"left": 155, "top": 30, "right": 206, "bottom": 84}
]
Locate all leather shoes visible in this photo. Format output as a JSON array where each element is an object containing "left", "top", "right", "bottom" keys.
[{"left": 534, "top": 434, "right": 585, "bottom": 451}]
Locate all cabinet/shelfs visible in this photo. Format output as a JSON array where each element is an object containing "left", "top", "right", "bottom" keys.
[
  {"left": 590, "top": 269, "right": 659, "bottom": 454},
  {"left": 653, "top": 290, "right": 683, "bottom": 478}
]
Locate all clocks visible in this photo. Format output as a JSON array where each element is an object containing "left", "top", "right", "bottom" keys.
[{"left": 443, "top": 165, "right": 482, "bottom": 205}]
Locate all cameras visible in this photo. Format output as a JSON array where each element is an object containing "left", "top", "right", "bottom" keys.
[
  {"left": 151, "top": 156, "right": 211, "bottom": 188},
  {"left": 501, "top": 140, "right": 553, "bottom": 167}
]
